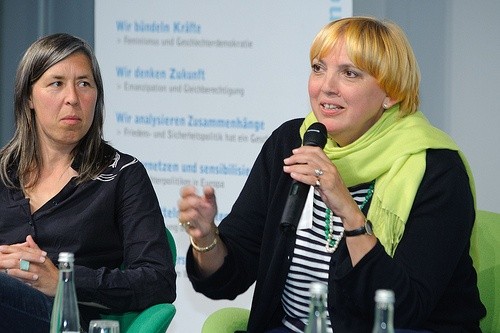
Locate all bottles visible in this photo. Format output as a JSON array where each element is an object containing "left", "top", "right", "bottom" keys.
[
  {"left": 50, "top": 251, "right": 80, "bottom": 333},
  {"left": 304, "top": 281, "right": 328, "bottom": 333},
  {"left": 372, "top": 289, "right": 395, "bottom": 333}
]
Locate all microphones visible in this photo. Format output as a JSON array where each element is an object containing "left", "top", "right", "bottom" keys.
[{"left": 279, "top": 122, "right": 327, "bottom": 234}]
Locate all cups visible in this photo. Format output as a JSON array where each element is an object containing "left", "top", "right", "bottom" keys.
[{"left": 88, "top": 320, "right": 120, "bottom": 333}]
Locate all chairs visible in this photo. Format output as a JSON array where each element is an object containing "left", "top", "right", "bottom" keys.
[
  {"left": 93, "top": 227, "right": 177, "bottom": 333},
  {"left": 201, "top": 209, "right": 500, "bottom": 332}
]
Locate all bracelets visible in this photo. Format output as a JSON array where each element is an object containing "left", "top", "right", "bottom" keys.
[{"left": 190, "top": 228, "right": 220, "bottom": 252}]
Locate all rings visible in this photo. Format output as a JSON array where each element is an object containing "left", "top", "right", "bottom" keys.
[
  {"left": 315, "top": 168, "right": 323, "bottom": 176},
  {"left": 179, "top": 222, "right": 191, "bottom": 228},
  {"left": 19, "top": 259, "right": 29, "bottom": 271},
  {"left": 4, "top": 268, "right": 7, "bottom": 274},
  {"left": 315, "top": 176, "right": 320, "bottom": 187}
]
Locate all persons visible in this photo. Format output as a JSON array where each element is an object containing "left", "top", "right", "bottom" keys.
[
  {"left": 0, "top": 32, "right": 177, "bottom": 333},
  {"left": 178, "top": 15, "right": 488, "bottom": 333}
]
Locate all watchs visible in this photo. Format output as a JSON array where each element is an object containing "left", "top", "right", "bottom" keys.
[{"left": 344, "top": 220, "right": 374, "bottom": 237}]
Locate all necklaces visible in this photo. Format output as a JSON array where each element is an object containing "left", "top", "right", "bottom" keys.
[
  {"left": 326, "top": 211, "right": 344, "bottom": 253},
  {"left": 325, "top": 179, "right": 375, "bottom": 245}
]
